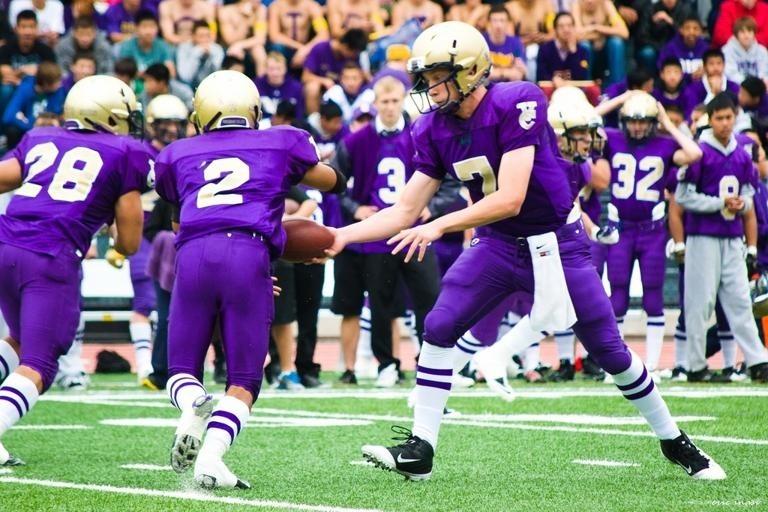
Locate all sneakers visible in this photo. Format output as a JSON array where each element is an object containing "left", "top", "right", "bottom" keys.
[
  {"left": 363, "top": 434, "right": 439, "bottom": 482},
  {"left": 56, "top": 368, "right": 91, "bottom": 392},
  {"left": 213, "top": 360, "right": 227, "bottom": 385},
  {"left": 170, "top": 394, "right": 214, "bottom": 474},
  {"left": 268, "top": 362, "right": 403, "bottom": 395},
  {"left": 451, "top": 367, "right": 478, "bottom": 391},
  {"left": 193, "top": 455, "right": 253, "bottom": 492},
  {"left": 647, "top": 361, "right": 768, "bottom": 386},
  {"left": 137, "top": 366, "right": 168, "bottom": 390},
  {"left": 657, "top": 427, "right": 727, "bottom": 482},
  {"left": 515, "top": 359, "right": 615, "bottom": 384}
]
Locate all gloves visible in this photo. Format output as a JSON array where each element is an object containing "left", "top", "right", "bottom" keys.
[{"left": 595, "top": 224, "right": 619, "bottom": 245}]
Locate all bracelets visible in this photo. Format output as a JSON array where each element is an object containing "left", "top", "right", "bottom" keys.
[{"left": 323, "top": 161, "right": 347, "bottom": 196}]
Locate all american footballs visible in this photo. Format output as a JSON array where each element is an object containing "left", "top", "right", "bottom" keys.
[{"left": 280, "top": 218, "right": 335, "bottom": 262}]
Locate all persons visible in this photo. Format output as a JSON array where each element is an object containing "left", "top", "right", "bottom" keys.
[
  {"left": 152, "top": 67, "right": 351, "bottom": 493},
  {"left": 1, "top": 2, "right": 768, "bottom": 389},
  {"left": 301, "top": 23, "right": 734, "bottom": 488},
  {"left": 0, "top": 72, "right": 155, "bottom": 471}
]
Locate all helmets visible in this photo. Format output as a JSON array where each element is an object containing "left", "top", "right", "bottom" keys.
[
  {"left": 61, "top": 74, "right": 145, "bottom": 139},
  {"left": 145, "top": 91, "right": 189, "bottom": 139},
  {"left": 189, "top": 68, "right": 264, "bottom": 134},
  {"left": 546, "top": 85, "right": 604, "bottom": 156},
  {"left": 406, "top": 19, "right": 494, "bottom": 111},
  {"left": 618, "top": 89, "right": 660, "bottom": 132}
]
